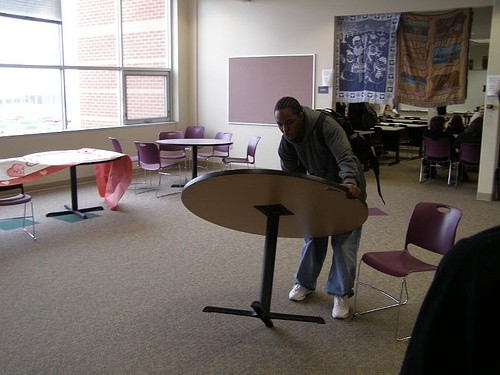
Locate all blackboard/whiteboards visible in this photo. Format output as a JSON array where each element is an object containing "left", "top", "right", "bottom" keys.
[{"left": 226, "top": 53, "right": 316, "bottom": 128}]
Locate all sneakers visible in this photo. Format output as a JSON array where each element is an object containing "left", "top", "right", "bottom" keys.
[
  {"left": 288, "top": 283, "right": 312, "bottom": 300},
  {"left": 332, "top": 294, "right": 350, "bottom": 318}
]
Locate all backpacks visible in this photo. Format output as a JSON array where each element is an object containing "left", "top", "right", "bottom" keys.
[{"left": 315, "top": 108, "right": 379, "bottom": 173}]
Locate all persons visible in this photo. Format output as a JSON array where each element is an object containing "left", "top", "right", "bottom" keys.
[
  {"left": 273, "top": 97, "right": 366, "bottom": 319},
  {"left": 422, "top": 106, "right": 485, "bottom": 183}
]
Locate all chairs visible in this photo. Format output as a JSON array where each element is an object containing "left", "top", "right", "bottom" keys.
[
  {"left": 0, "top": 125, "right": 261, "bottom": 240},
  {"left": 353, "top": 201, "right": 462, "bottom": 340},
  {"left": 365, "top": 115, "right": 482, "bottom": 187}
]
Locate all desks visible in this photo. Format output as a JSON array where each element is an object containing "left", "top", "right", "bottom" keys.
[
  {"left": 156, "top": 139, "right": 233, "bottom": 188},
  {"left": 374, "top": 126, "right": 405, "bottom": 166},
  {"left": 0, "top": 147, "right": 133, "bottom": 219},
  {"left": 182, "top": 168, "right": 368, "bottom": 327},
  {"left": 379, "top": 122, "right": 428, "bottom": 160},
  {"left": 385, "top": 118, "right": 428, "bottom": 124}
]
